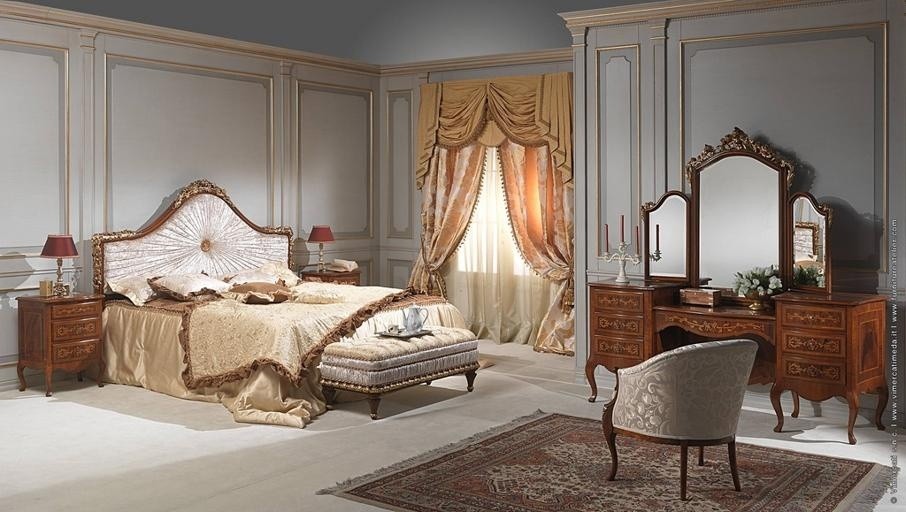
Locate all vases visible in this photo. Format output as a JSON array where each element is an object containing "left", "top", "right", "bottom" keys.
[{"left": 748, "top": 296, "right": 768, "bottom": 316}]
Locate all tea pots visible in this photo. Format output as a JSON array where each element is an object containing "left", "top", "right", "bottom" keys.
[{"left": 399, "top": 301, "right": 428, "bottom": 334}]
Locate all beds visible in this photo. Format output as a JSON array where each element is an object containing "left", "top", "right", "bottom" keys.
[{"left": 92, "top": 179, "right": 465, "bottom": 430}]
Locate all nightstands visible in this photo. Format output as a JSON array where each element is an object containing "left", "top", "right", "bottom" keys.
[
  {"left": 15, "top": 292, "right": 106, "bottom": 399},
  {"left": 301, "top": 269, "right": 361, "bottom": 287}
]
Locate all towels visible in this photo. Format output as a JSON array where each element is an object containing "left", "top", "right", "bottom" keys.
[{"left": 328, "top": 256, "right": 359, "bottom": 272}]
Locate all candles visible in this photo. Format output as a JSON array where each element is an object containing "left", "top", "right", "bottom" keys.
[
  {"left": 635, "top": 225, "right": 639, "bottom": 254},
  {"left": 656, "top": 224, "right": 660, "bottom": 252},
  {"left": 604, "top": 224, "right": 608, "bottom": 254},
  {"left": 620, "top": 215, "right": 624, "bottom": 242}
]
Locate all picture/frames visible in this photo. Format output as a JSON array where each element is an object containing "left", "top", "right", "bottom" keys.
[{"left": 796, "top": 222, "right": 816, "bottom": 261}]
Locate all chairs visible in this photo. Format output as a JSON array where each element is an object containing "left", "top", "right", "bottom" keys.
[{"left": 602, "top": 336, "right": 759, "bottom": 502}]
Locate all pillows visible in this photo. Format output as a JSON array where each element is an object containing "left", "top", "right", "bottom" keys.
[{"left": 105, "top": 263, "right": 301, "bottom": 304}]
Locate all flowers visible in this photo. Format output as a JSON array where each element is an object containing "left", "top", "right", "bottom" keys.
[
  {"left": 795, "top": 266, "right": 823, "bottom": 286},
  {"left": 733, "top": 263, "right": 783, "bottom": 311}
]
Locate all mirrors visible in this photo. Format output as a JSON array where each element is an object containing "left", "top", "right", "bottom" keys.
[
  {"left": 788, "top": 190, "right": 834, "bottom": 294},
  {"left": 639, "top": 189, "right": 689, "bottom": 281},
  {"left": 684, "top": 124, "right": 796, "bottom": 291}
]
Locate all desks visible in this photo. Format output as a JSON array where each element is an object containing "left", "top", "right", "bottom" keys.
[{"left": 654, "top": 300, "right": 776, "bottom": 354}]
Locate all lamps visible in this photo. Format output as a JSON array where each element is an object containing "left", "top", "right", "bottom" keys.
[
  {"left": 40, "top": 233, "right": 80, "bottom": 298},
  {"left": 305, "top": 224, "right": 336, "bottom": 272}
]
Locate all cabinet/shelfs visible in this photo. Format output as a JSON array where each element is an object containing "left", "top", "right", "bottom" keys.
[
  {"left": 584, "top": 277, "right": 683, "bottom": 404},
  {"left": 770, "top": 290, "right": 890, "bottom": 445}
]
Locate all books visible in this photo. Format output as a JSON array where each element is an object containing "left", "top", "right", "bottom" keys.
[
  {"left": 39, "top": 280, "right": 53, "bottom": 298},
  {"left": 326, "top": 258, "right": 359, "bottom": 272}
]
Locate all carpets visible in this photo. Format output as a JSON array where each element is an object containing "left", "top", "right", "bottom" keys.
[{"left": 317, "top": 409, "right": 898, "bottom": 512}]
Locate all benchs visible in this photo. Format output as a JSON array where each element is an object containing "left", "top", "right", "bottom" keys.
[{"left": 318, "top": 324, "right": 479, "bottom": 421}]
